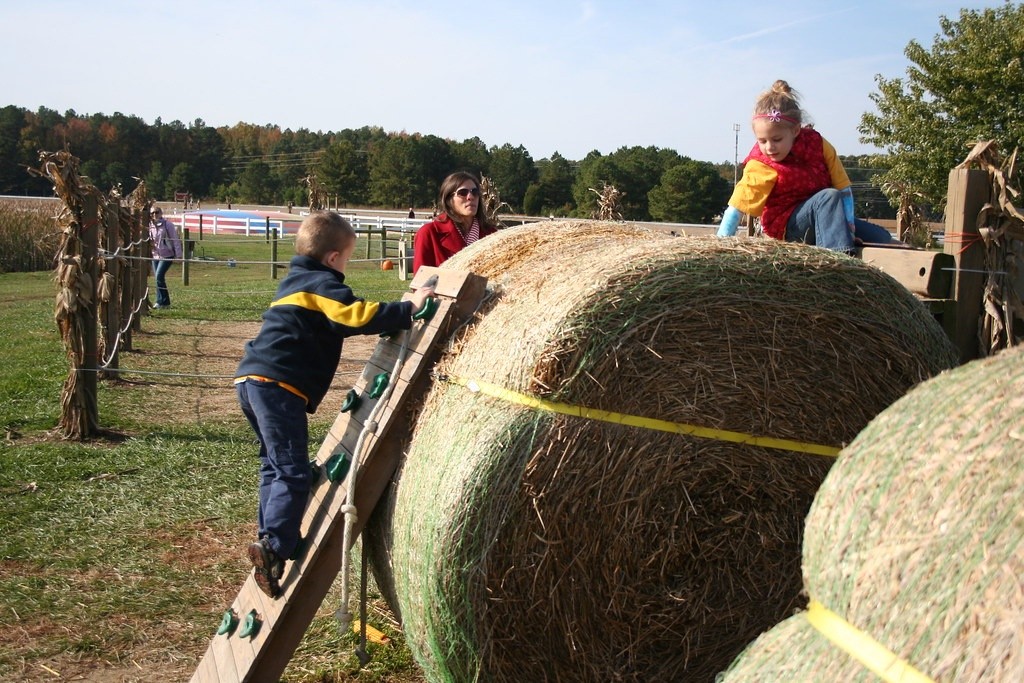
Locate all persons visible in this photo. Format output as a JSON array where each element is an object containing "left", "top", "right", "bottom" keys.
[
  {"left": 408, "top": 208, "right": 416, "bottom": 225},
  {"left": 411, "top": 171, "right": 498, "bottom": 292},
  {"left": 233, "top": 211, "right": 437, "bottom": 600},
  {"left": 150, "top": 207, "right": 182, "bottom": 308},
  {"left": 714, "top": 80, "right": 892, "bottom": 256}
]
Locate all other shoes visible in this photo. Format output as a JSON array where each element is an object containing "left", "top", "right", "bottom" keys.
[{"left": 150, "top": 303, "right": 170, "bottom": 309}]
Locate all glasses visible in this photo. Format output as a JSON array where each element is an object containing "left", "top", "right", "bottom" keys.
[
  {"left": 450, "top": 188, "right": 479, "bottom": 197},
  {"left": 150, "top": 210, "right": 160, "bottom": 215}
]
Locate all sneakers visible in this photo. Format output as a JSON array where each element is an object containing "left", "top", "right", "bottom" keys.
[{"left": 247, "top": 534, "right": 285, "bottom": 601}]
